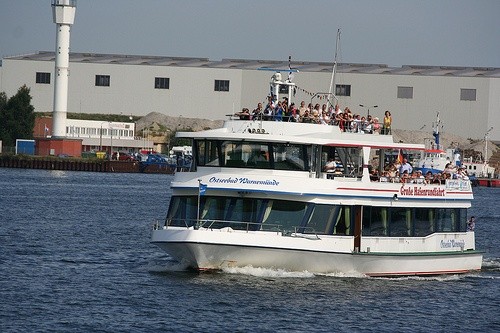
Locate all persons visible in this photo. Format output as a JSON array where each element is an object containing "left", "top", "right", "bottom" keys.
[{"left": 133, "top": 69, "right": 476, "bottom": 233}]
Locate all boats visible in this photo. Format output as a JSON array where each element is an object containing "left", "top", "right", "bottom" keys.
[
  {"left": 412, "top": 112, "right": 500, "bottom": 188},
  {"left": 150, "top": 55, "right": 486, "bottom": 277}
]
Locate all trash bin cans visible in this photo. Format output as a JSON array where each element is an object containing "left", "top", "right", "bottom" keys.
[{"left": 82, "top": 151, "right": 108, "bottom": 158}]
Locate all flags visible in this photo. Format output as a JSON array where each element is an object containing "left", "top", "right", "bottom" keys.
[
  {"left": 397, "top": 149, "right": 404, "bottom": 164},
  {"left": 199, "top": 183, "right": 207, "bottom": 195}
]
